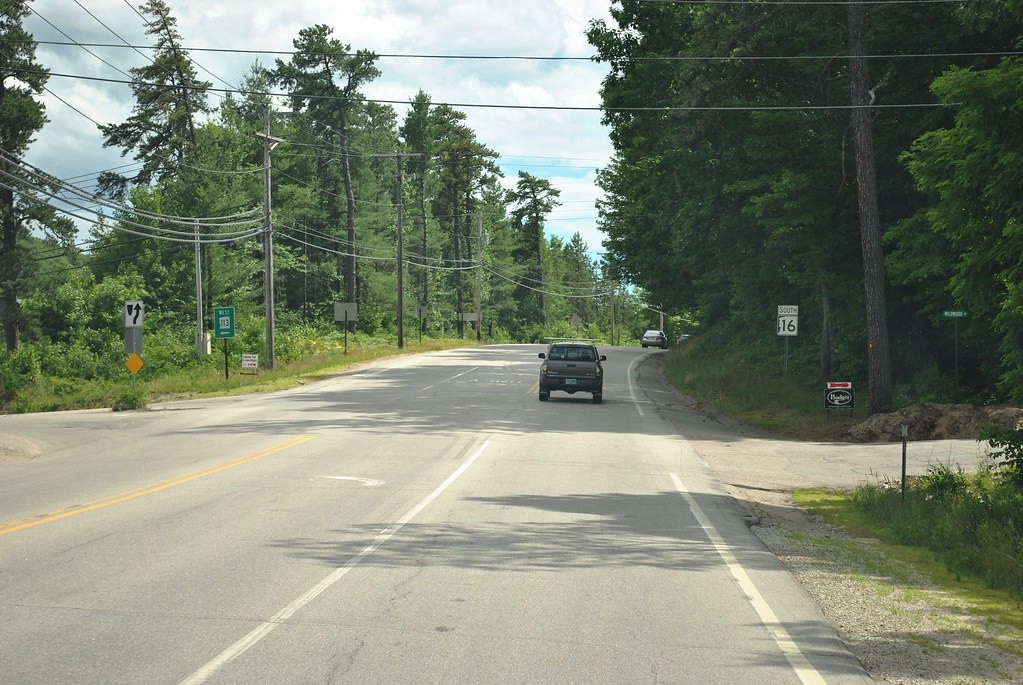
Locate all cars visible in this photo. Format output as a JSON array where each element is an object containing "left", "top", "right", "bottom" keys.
[
  {"left": 641, "top": 329, "right": 667, "bottom": 349},
  {"left": 538, "top": 342, "right": 607, "bottom": 404}
]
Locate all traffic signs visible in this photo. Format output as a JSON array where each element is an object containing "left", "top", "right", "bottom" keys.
[{"left": 214, "top": 308, "right": 235, "bottom": 339}]
